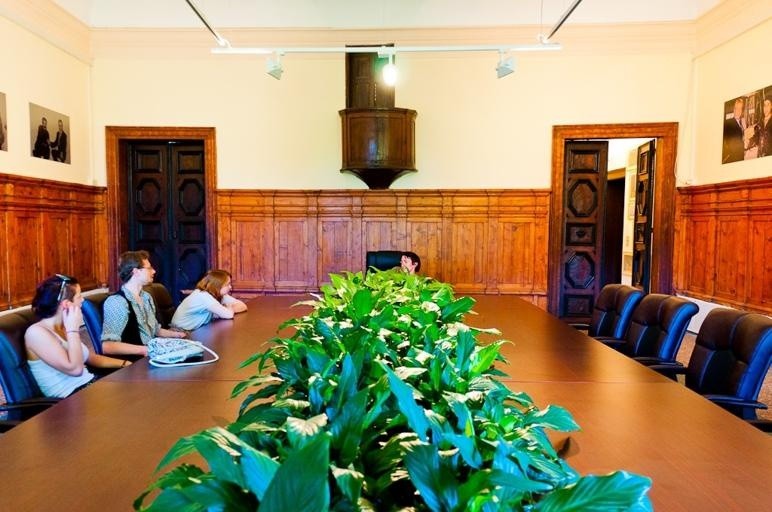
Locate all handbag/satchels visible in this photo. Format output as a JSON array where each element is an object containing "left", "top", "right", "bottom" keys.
[{"left": 147, "top": 338, "right": 219, "bottom": 368}]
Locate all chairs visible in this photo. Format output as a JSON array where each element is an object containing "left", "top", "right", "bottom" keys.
[
  {"left": 599, "top": 293, "right": 699, "bottom": 382},
  {"left": 643, "top": 307, "right": 772, "bottom": 435},
  {"left": 557, "top": 280, "right": 646, "bottom": 356},
  {"left": 365, "top": 250, "right": 418, "bottom": 284},
  {"left": 79, "top": 293, "right": 121, "bottom": 362},
  {"left": 144, "top": 280, "right": 179, "bottom": 331},
  {"left": 0, "top": 301, "right": 57, "bottom": 421}
]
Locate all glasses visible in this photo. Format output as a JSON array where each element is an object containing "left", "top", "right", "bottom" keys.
[{"left": 55, "top": 273, "right": 77, "bottom": 302}]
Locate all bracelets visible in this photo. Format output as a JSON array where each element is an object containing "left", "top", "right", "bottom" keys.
[{"left": 119, "top": 359, "right": 128, "bottom": 369}]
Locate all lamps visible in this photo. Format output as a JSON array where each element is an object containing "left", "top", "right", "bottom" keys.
[{"left": 262, "top": 50, "right": 520, "bottom": 86}]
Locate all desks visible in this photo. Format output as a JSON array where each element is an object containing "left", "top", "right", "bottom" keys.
[{"left": 0, "top": 293, "right": 772, "bottom": 511}]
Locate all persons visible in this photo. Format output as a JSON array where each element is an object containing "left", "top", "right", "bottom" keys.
[
  {"left": 100, "top": 249, "right": 190, "bottom": 356},
  {"left": 748, "top": 95, "right": 771, "bottom": 157},
  {"left": 34, "top": 117, "right": 51, "bottom": 159},
  {"left": 167, "top": 269, "right": 249, "bottom": 333},
  {"left": 397, "top": 250, "right": 422, "bottom": 275},
  {"left": 22, "top": 274, "right": 134, "bottom": 399},
  {"left": 721, "top": 98, "right": 746, "bottom": 162},
  {"left": 51, "top": 120, "right": 68, "bottom": 162}
]
